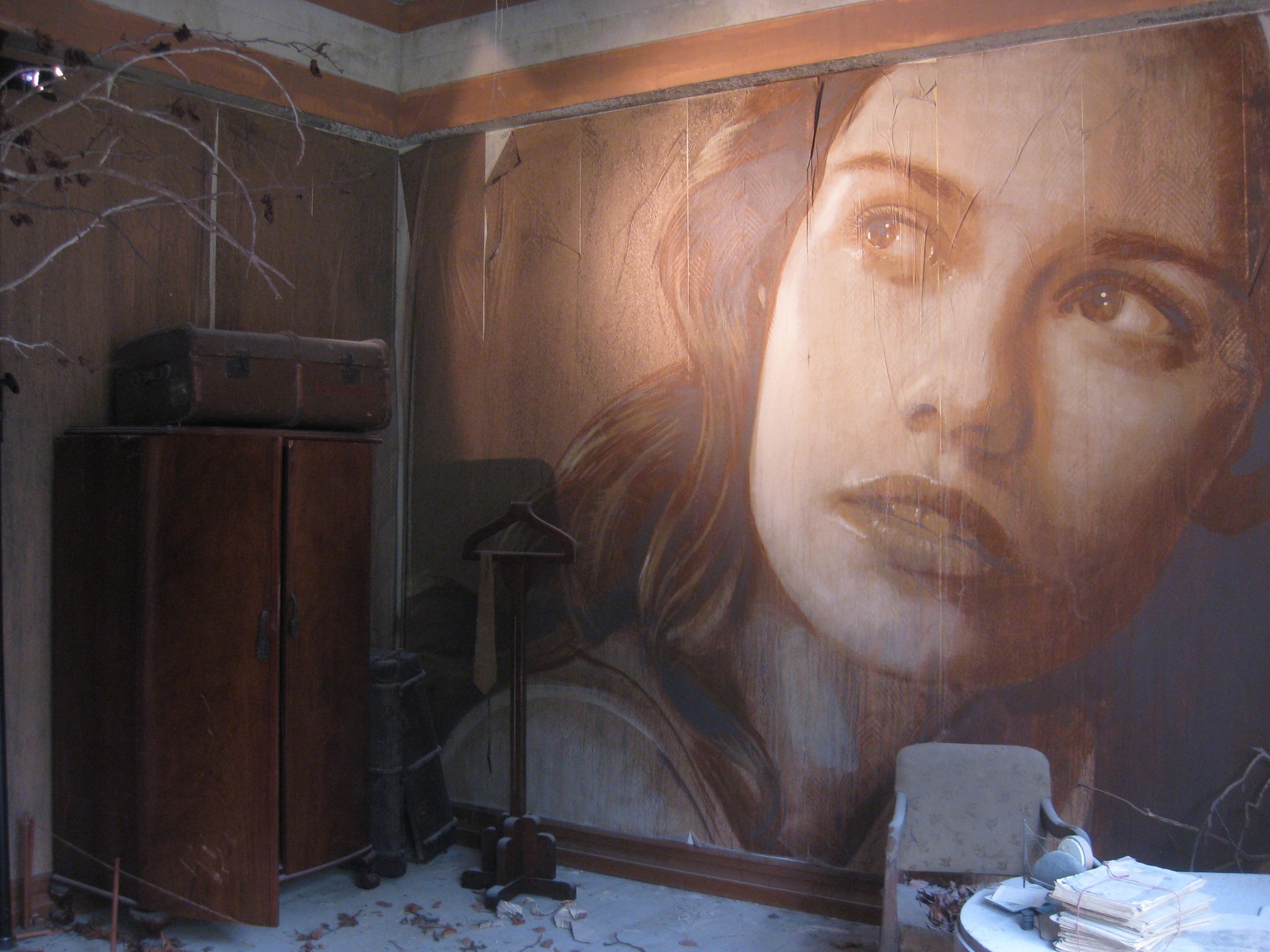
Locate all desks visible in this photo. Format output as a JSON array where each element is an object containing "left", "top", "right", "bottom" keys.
[{"left": 956, "top": 873, "right": 1270, "bottom": 952}]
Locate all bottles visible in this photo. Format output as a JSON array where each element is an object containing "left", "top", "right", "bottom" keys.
[{"left": 1021, "top": 909, "right": 1034, "bottom": 930}]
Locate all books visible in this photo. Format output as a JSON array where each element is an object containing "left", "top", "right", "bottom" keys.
[{"left": 1048, "top": 856, "right": 1219, "bottom": 952}]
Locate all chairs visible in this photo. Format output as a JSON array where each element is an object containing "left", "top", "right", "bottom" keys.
[{"left": 881, "top": 741, "right": 1092, "bottom": 952}]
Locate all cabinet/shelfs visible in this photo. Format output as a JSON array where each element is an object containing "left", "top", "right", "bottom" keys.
[{"left": 49, "top": 428, "right": 385, "bottom": 928}]
